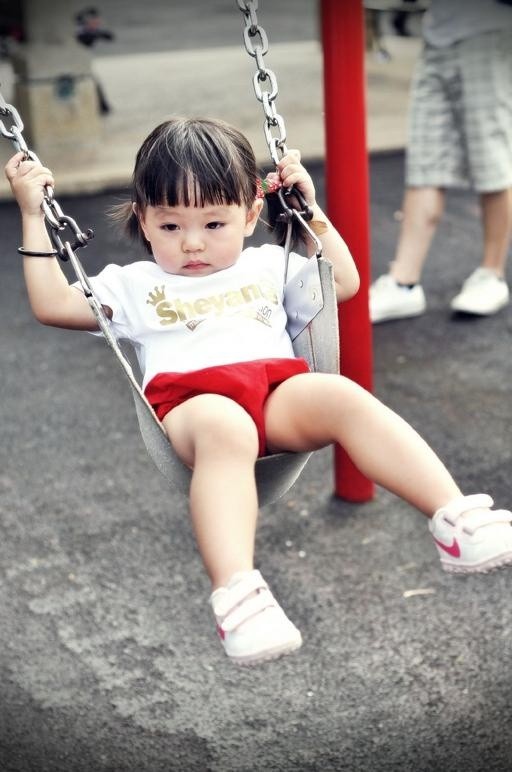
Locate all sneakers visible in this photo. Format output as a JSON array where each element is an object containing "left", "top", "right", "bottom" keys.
[
  {"left": 208, "top": 569, "right": 303, "bottom": 665},
  {"left": 449, "top": 267, "right": 509, "bottom": 315},
  {"left": 427, "top": 494, "right": 512, "bottom": 573},
  {"left": 369, "top": 274, "right": 425, "bottom": 324}
]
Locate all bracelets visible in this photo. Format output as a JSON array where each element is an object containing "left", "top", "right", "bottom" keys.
[{"left": 15, "top": 244, "right": 61, "bottom": 257}]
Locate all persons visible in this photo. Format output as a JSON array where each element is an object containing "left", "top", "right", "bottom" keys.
[
  {"left": 2, "top": 116, "right": 512, "bottom": 668},
  {"left": 369, "top": 1, "right": 512, "bottom": 327}
]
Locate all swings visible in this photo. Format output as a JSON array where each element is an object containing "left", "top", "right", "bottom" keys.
[{"left": 2, "top": 0, "right": 342, "bottom": 506}]
